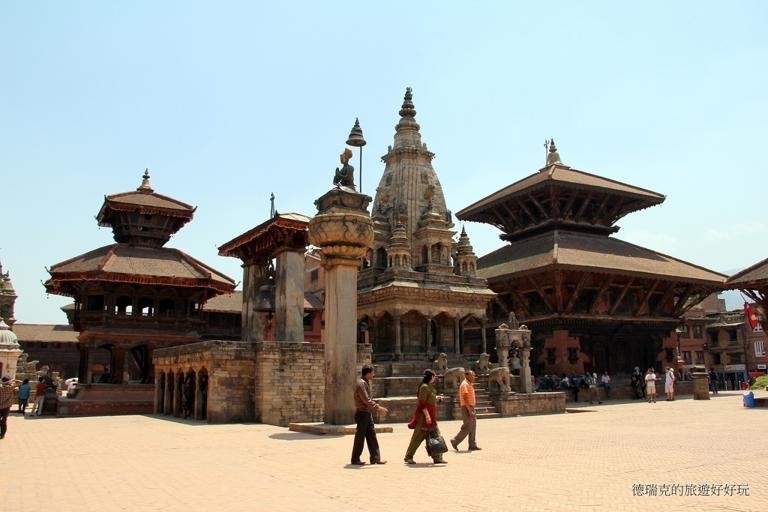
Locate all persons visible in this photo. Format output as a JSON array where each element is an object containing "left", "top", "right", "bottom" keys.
[
  {"left": 333, "top": 148, "right": 355, "bottom": 190},
  {"left": 452, "top": 370, "right": 483, "bottom": 451},
  {"left": 404, "top": 369, "right": 449, "bottom": 463},
  {"left": 351, "top": 363, "right": 388, "bottom": 465},
  {"left": 0, "top": 374, "right": 47, "bottom": 439},
  {"left": 537, "top": 365, "right": 719, "bottom": 403}
]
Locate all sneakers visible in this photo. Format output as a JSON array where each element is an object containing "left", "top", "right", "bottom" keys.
[
  {"left": 469, "top": 447, "right": 482, "bottom": 450},
  {"left": 371, "top": 461, "right": 386, "bottom": 464},
  {"left": 405, "top": 458, "right": 417, "bottom": 464},
  {"left": 354, "top": 461, "right": 365, "bottom": 465},
  {"left": 434, "top": 460, "right": 448, "bottom": 464},
  {"left": 451, "top": 440, "right": 458, "bottom": 451}
]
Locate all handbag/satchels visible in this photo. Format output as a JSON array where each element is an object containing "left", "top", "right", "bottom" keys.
[{"left": 426, "top": 436, "right": 448, "bottom": 456}]
